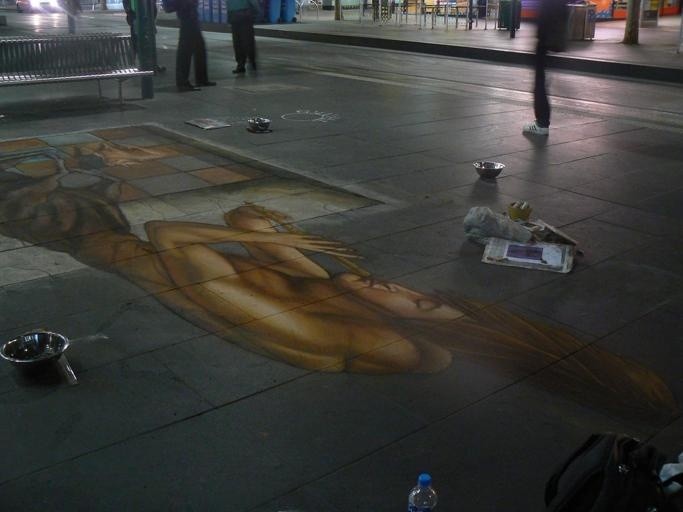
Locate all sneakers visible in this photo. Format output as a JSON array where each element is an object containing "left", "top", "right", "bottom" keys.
[
  {"left": 195, "top": 80, "right": 217, "bottom": 86},
  {"left": 232, "top": 65, "right": 247, "bottom": 73},
  {"left": 175, "top": 83, "right": 203, "bottom": 92},
  {"left": 521, "top": 121, "right": 550, "bottom": 136}
]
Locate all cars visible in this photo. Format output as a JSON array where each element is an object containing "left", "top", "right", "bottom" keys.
[{"left": 13, "top": 1, "right": 57, "bottom": 13}]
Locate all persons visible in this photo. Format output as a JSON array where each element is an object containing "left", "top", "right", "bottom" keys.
[
  {"left": 0, "top": 168, "right": 676, "bottom": 426},
  {"left": 161, "top": 0, "right": 216, "bottom": 91},
  {"left": 225, "top": 0, "right": 258, "bottom": 73},
  {"left": 122, "top": 0, "right": 166, "bottom": 73},
  {"left": 521, "top": 1, "right": 567, "bottom": 136}
]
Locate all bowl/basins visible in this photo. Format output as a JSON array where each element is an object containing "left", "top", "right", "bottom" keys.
[
  {"left": 1, "top": 331, "right": 69, "bottom": 372},
  {"left": 473, "top": 161, "right": 506, "bottom": 180},
  {"left": 247, "top": 118, "right": 271, "bottom": 132},
  {"left": 509, "top": 201, "right": 532, "bottom": 223}
]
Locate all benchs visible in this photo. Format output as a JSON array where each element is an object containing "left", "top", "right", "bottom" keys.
[{"left": 0, "top": 32, "right": 156, "bottom": 118}]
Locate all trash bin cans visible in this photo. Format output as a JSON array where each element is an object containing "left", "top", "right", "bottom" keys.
[
  {"left": 263, "top": 0, "right": 297, "bottom": 24},
  {"left": 565, "top": 0, "right": 597, "bottom": 41},
  {"left": 498, "top": 0, "right": 521, "bottom": 28}
]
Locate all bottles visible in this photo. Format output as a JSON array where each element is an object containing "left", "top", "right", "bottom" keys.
[{"left": 407, "top": 474, "right": 438, "bottom": 511}]
